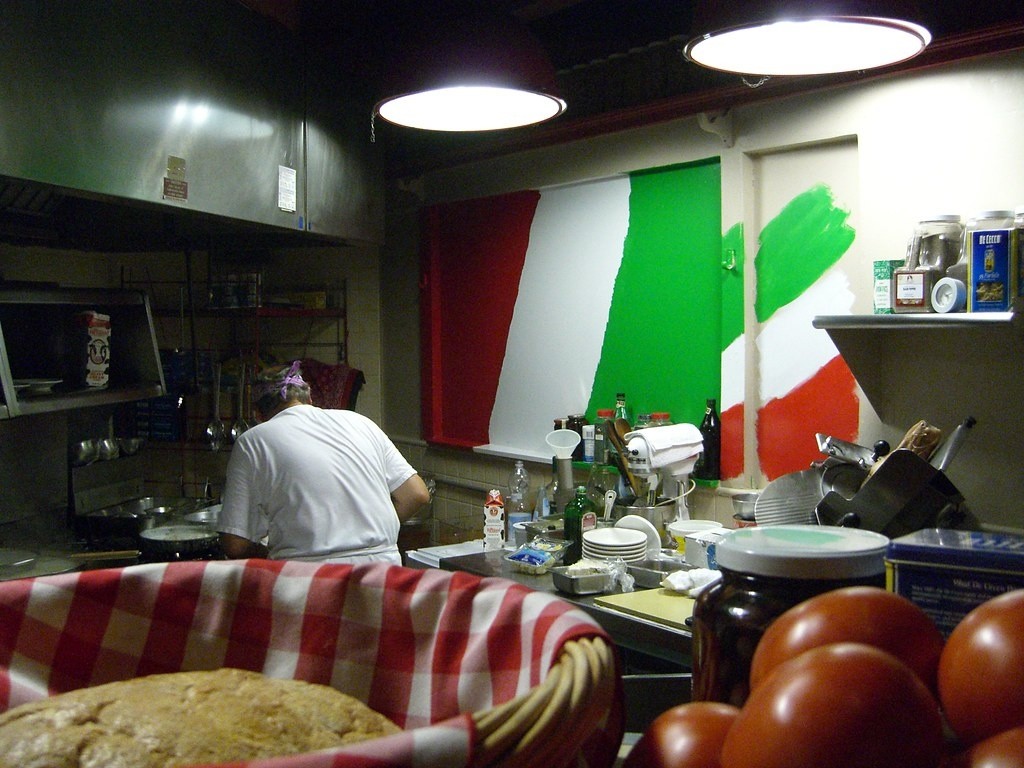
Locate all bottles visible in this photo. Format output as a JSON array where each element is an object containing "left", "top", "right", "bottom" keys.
[
  {"left": 690, "top": 524, "right": 890, "bottom": 707},
  {"left": 505, "top": 461, "right": 617, "bottom": 567},
  {"left": 892, "top": 210, "right": 1024, "bottom": 313},
  {"left": 553, "top": 392, "right": 720, "bottom": 481}
]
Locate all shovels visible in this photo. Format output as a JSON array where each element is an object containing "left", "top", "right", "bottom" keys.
[
  {"left": 614, "top": 418, "right": 632, "bottom": 445},
  {"left": 604, "top": 420, "right": 640, "bottom": 498}
]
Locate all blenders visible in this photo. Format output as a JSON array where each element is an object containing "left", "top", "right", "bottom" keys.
[{"left": 627, "top": 423, "right": 705, "bottom": 522}]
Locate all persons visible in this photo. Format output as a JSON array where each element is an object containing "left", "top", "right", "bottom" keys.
[{"left": 218, "top": 366, "right": 429, "bottom": 567}]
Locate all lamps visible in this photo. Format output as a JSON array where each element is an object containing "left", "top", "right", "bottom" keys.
[
  {"left": 684, "top": 0, "right": 932, "bottom": 78},
  {"left": 374, "top": 1, "right": 569, "bottom": 134}
]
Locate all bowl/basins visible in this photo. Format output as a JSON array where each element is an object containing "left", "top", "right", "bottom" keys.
[
  {"left": 733, "top": 494, "right": 761, "bottom": 519},
  {"left": 68, "top": 437, "right": 142, "bottom": 466}
]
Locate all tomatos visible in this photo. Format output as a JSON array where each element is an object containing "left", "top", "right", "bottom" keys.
[{"left": 611, "top": 585, "right": 1023, "bottom": 767}]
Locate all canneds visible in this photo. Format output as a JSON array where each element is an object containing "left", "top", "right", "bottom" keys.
[{"left": 964, "top": 210, "right": 1024, "bottom": 315}]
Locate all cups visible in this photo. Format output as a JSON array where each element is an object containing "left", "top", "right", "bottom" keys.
[{"left": 668, "top": 520, "right": 723, "bottom": 553}]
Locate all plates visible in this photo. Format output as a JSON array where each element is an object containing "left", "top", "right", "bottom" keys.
[
  {"left": 13, "top": 377, "right": 63, "bottom": 398},
  {"left": 581, "top": 514, "right": 662, "bottom": 566}
]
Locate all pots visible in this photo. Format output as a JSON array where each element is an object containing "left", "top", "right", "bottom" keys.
[{"left": 139, "top": 510, "right": 220, "bottom": 554}]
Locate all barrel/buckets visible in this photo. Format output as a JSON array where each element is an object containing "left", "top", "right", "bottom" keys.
[{"left": 614, "top": 495, "right": 677, "bottom": 547}]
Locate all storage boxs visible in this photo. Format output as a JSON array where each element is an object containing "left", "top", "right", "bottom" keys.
[{"left": 885, "top": 528, "right": 1023, "bottom": 637}]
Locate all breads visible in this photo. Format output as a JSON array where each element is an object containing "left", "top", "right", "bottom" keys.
[{"left": 0, "top": 668, "right": 405, "bottom": 768}]
